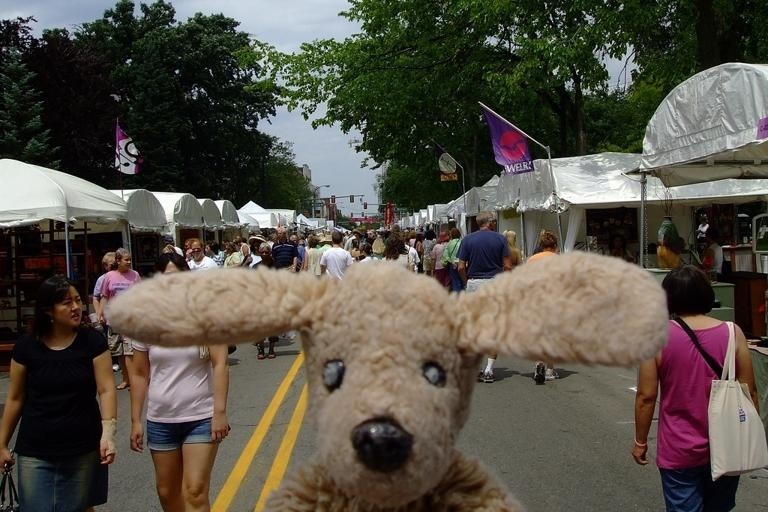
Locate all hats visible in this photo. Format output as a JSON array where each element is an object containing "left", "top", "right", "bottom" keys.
[
  {"left": 320, "top": 234, "right": 333, "bottom": 242},
  {"left": 436, "top": 231, "right": 450, "bottom": 242}
]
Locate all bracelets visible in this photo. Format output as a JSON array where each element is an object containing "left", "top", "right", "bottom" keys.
[{"left": 633, "top": 438, "right": 648, "bottom": 447}]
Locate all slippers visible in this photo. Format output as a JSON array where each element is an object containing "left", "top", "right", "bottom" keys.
[{"left": 116, "top": 379, "right": 130, "bottom": 391}]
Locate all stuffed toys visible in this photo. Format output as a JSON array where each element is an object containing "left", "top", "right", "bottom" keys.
[{"left": 102, "top": 250, "right": 671, "bottom": 512}]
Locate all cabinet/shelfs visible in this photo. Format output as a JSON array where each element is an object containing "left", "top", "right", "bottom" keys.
[{"left": 0, "top": 221, "right": 90, "bottom": 372}]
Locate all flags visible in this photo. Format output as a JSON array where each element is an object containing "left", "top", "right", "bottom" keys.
[
  {"left": 484, "top": 109, "right": 537, "bottom": 177},
  {"left": 113, "top": 125, "right": 144, "bottom": 177}
]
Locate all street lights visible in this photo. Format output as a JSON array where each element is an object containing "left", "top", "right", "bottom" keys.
[{"left": 312, "top": 184, "right": 331, "bottom": 218}]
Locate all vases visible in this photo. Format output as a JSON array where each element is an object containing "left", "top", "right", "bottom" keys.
[{"left": 656, "top": 215, "right": 680, "bottom": 269}]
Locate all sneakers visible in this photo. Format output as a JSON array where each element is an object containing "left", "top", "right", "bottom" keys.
[{"left": 477, "top": 361, "right": 564, "bottom": 385}]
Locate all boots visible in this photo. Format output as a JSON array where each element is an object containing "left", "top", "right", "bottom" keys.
[{"left": 254, "top": 342, "right": 278, "bottom": 360}]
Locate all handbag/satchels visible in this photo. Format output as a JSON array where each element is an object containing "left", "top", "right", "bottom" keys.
[{"left": 708, "top": 379, "right": 767, "bottom": 480}]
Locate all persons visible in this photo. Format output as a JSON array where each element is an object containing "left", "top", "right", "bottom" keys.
[
  {"left": 527, "top": 228, "right": 562, "bottom": 385},
  {"left": 0, "top": 274, "right": 118, "bottom": 512},
  {"left": 697, "top": 216, "right": 709, "bottom": 242},
  {"left": 456, "top": 211, "right": 512, "bottom": 384},
  {"left": 703, "top": 227, "right": 724, "bottom": 281},
  {"left": 629, "top": 263, "right": 762, "bottom": 511},
  {"left": 98, "top": 247, "right": 145, "bottom": 392},
  {"left": 129, "top": 251, "right": 230, "bottom": 512},
  {"left": 656, "top": 232, "right": 684, "bottom": 268},
  {"left": 93, "top": 250, "right": 121, "bottom": 372},
  {"left": 161, "top": 223, "right": 525, "bottom": 360}
]
